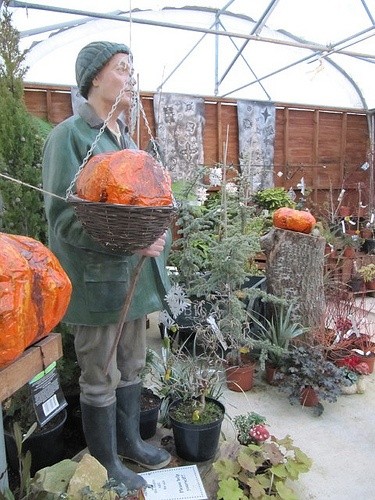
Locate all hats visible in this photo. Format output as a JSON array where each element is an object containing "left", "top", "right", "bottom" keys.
[{"left": 75, "top": 41, "right": 133, "bottom": 99}]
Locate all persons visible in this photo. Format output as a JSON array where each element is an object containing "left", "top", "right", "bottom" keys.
[{"left": 40, "top": 40, "right": 173, "bottom": 493}]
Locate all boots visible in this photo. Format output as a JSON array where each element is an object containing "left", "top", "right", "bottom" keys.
[
  {"left": 81, "top": 397, "right": 147, "bottom": 491},
  {"left": 116, "top": 381, "right": 172, "bottom": 468}
]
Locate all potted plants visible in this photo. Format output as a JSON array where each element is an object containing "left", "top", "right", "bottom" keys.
[
  {"left": 284, "top": 347, "right": 352, "bottom": 419},
  {"left": 137, "top": 349, "right": 161, "bottom": 438},
  {"left": 234, "top": 411, "right": 268, "bottom": 449},
  {"left": 160, "top": 338, "right": 240, "bottom": 463},
  {"left": 243, "top": 303, "right": 311, "bottom": 386},
  {"left": 4, "top": 384, "right": 66, "bottom": 469},
  {"left": 212, "top": 434, "right": 313, "bottom": 500},
  {"left": 181, "top": 222, "right": 291, "bottom": 393},
  {"left": 174, "top": 171, "right": 303, "bottom": 273},
  {"left": 0, "top": 10, "right": 86, "bottom": 430}
]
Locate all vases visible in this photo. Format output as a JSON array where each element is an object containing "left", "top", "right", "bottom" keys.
[{"left": 321, "top": 203, "right": 375, "bottom": 394}]
